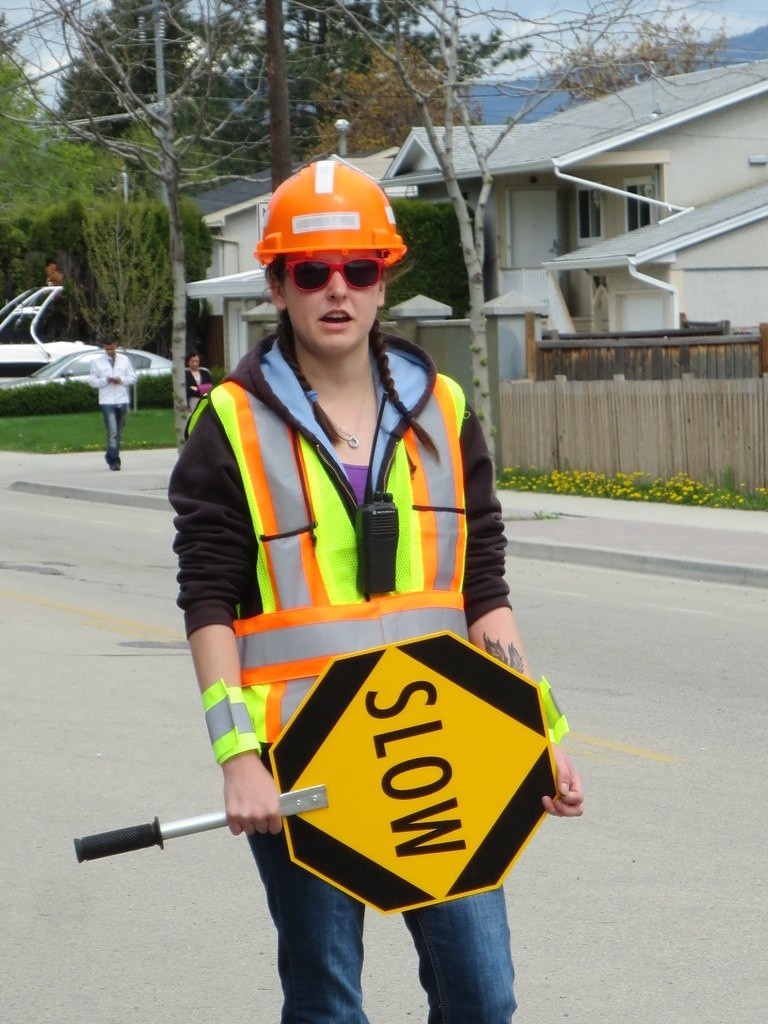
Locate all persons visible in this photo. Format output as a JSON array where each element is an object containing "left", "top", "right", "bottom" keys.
[
  {"left": 183, "top": 350, "right": 213, "bottom": 419},
  {"left": 88, "top": 335, "right": 137, "bottom": 470},
  {"left": 167, "top": 153, "right": 587, "bottom": 1023}
]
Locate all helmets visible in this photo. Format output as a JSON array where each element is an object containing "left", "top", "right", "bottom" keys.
[{"left": 255, "top": 155, "right": 409, "bottom": 263}]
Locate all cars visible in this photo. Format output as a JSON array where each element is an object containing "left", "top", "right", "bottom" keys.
[
  {"left": 0, "top": 284, "right": 100, "bottom": 387},
  {"left": 0, "top": 344, "right": 209, "bottom": 389}
]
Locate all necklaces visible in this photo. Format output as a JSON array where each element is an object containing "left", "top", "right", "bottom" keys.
[{"left": 323, "top": 362, "right": 375, "bottom": 448}]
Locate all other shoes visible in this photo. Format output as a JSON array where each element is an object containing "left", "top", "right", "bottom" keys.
[{"left": 109, "top": 462, "right": 119, "bottom": 471}]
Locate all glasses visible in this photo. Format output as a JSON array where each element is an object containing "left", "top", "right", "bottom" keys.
[{"left": 280, "top": 256, "right": 385, "bottom": 291}]
